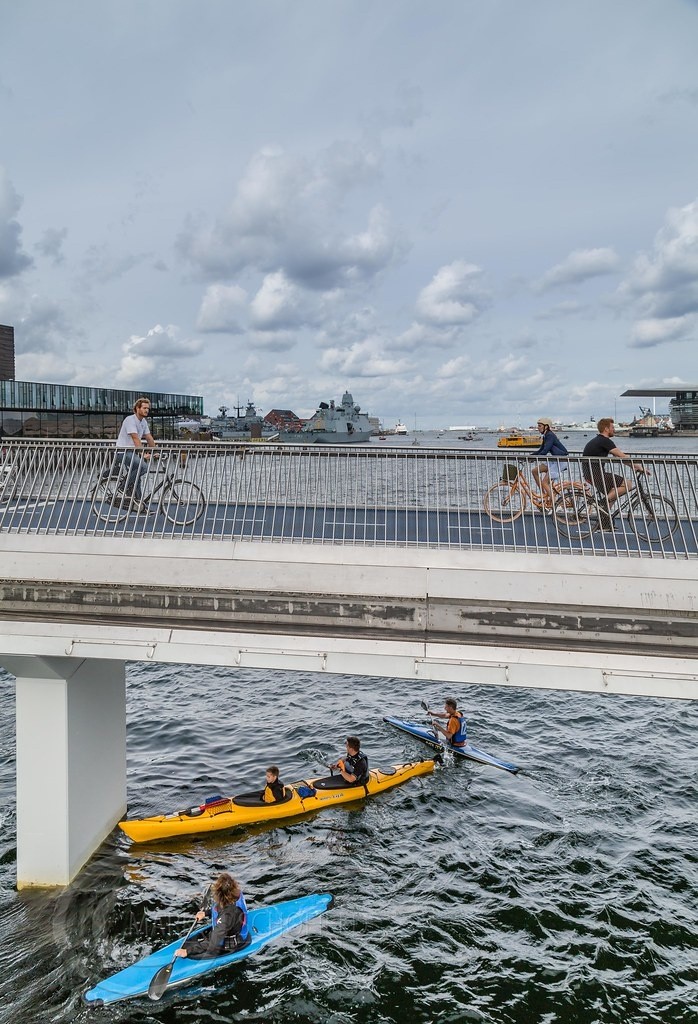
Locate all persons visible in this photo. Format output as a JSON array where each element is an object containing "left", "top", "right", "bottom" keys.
[
  {"left": 114, "top": 399, "right": 161, "bottom": 515},
  {"left": 261, "top": 766, "right": 286, "bottom": 803},
  {"left": 332, "top": 736, "right": 369, "bottom": 786},
  {"left": 173, "top": 873, "right": 249, "bottom": 959},
  {"left": 427, "top": 699, "right": 467, "bottom": 746},
  {"left": 582, "top": 419, "right": 652, "bottom": 532},
  {"left": 523, "top": 418, "right": 569, "bottom": 516}
]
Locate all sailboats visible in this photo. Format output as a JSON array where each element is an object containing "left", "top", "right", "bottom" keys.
[{"left": 413, "top": 413, "right": 421, "bottom": 433}]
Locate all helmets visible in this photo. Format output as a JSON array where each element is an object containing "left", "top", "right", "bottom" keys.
[{"left": 537, "top": 418, "right": 552, "bottom": 428}]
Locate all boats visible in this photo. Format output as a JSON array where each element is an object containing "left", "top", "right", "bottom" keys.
[
  {"left": 437, "top": 429, "right": 483, "bottom": 442},
  {"left": 379, "top": 437, "right": 387, "bottom": 440},
  {"left": 395, "top": 422, "right": 408, "bottom": 435},
  {"left": 191, "top": 390, "right": 377, "bottom": 444},
  {"left": 497, "top": 431, "right": 543, "bottom": 448},
  {"left": 381, "top": 717, "right": 521, "bottom": 774},
  {"left": 117, "top": 759, "right": 435, "bottom": 845},
  {"left": 81, "top": 892, "right": 337, "bottom": 1007}
]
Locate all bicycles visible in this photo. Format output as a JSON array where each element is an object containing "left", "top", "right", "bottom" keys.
[
  {"left": 0, "top": 464, "right": 18, "bottom": 505},
  {"left": 552, "top": 468, "right": 679, "bottom": 543},
  {"left": 90, "top": 451, "right": 205, "bottom": 526},
  {"left": 482, "top": 459, "right": 592, "bottom": 526}
]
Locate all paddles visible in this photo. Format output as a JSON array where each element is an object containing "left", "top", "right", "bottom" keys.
[
  {"left": 147, "top": 882, "right": 212, "bottom": 1001},
  {"left": 421, "top": 700, "right": 439, "bottom": 740},
  {"left": 330, "top": 760, "right": 345, "bottom": 773}
]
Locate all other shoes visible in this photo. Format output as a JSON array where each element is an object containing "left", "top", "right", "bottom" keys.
[
  {"left": 144, "top": 507, "right": 154, "bottom": 515},
  {"left": 597, "top": 526, "right": 619, "bottom": 532},
  {"left": 118, "top": 488, "right": 136, "bottom": 499},
  {"left": 598, "top": 501, "right": 606, "bottom": 517},
  {"left": 546, "top": 498, "right": 556, "bottom": 508}
]
